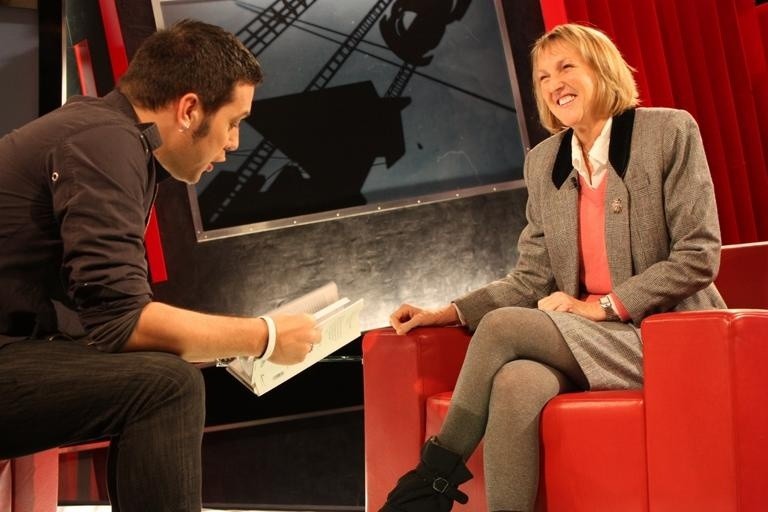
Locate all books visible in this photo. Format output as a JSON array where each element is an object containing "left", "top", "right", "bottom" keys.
[{"left": 219, "top": 278, "right": 364, "bottom": 398}]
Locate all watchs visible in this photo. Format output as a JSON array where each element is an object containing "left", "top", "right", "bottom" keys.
[{"left": 600, "top": 295, "right": 614, "bottom": 321}]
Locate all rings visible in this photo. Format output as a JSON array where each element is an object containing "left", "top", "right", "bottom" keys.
[{"left": 310, "top": 344, "right": 313, "bottom": 354}]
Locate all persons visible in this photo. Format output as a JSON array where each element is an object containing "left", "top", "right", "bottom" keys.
[
  {"left": 0, "top": 17, "right": 321, "bottom": 512},
  {"left": 378, "top": 21, "right": 729, "bottom": 512}
]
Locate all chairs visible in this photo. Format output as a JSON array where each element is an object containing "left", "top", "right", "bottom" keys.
[{"left": 364, "top": 239, "right": 767, "bottom": 512}]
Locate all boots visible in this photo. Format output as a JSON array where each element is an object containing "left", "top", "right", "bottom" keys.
[{"left": 377, "top": 436, "right": 472, "bottom": 512}]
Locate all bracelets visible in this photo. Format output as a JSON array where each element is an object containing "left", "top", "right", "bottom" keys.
[{"left": 257, "top": 314, "right": 275, "bottom": 364}]
